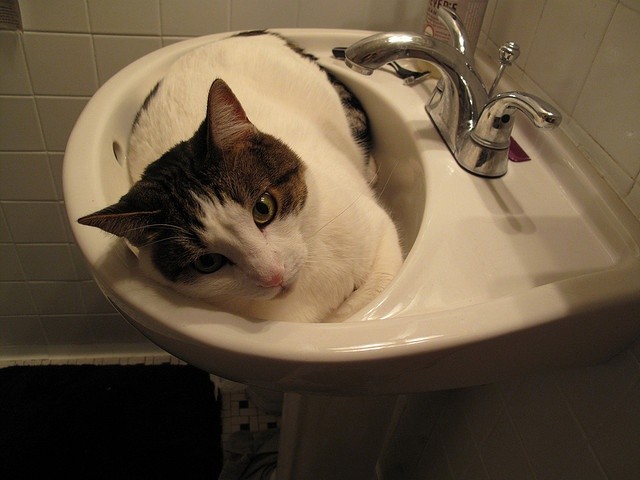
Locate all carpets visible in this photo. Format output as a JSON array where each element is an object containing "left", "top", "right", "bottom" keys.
[{"left": 0, "top": 364, "right": 223, "bottom": 479}]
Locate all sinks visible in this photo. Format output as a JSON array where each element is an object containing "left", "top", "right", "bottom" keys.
[{"left": 96, "top": 41, "right": 426, "bottom": 324}]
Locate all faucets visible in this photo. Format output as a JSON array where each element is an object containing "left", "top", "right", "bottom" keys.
[{"left": 343, "top": 30, "right": 491, "bottom": 132}]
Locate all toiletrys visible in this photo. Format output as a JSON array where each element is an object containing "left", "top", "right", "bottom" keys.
[{"left": 417, "top": 0, "right": 489, "bottom": 79}]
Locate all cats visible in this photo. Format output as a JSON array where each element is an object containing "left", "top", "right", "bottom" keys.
[{"left": 76, "top": 28, "right": 404, "bottom": 324}]
